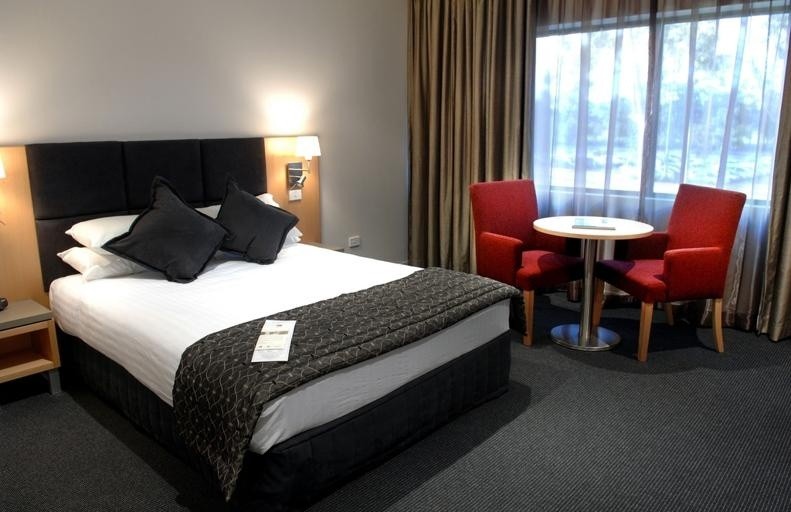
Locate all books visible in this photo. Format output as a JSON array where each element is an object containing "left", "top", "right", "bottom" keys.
[{"left": 572, "top": 213, "right": 617, "bottom": 231}]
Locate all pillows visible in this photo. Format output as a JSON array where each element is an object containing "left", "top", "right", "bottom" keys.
[{"left": 56, "top": 174, "right": 306, "bottom": 281}]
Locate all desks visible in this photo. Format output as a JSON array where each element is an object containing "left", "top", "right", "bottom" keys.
[{"left": 534, "top": 215, "right": 653, "bottom": 353}]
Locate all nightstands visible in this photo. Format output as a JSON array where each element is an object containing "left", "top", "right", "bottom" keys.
[{"left": 0, "top": 299, "right": 64, "bottom": 398}]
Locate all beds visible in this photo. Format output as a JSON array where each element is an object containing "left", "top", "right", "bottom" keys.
[{"left": 47, "top": 242, "right": 516, "bottom": 511}]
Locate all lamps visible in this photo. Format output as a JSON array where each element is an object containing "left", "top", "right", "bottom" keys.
[{"left": 286, "top": 135, "right": 322, "bottom": 201}]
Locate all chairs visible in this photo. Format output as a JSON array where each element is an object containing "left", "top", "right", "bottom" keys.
[
  {"left": 470, "top": 181, "right": 603, "bottom": 348},
  {"left": 591, "top": 184, "right": 747, "bottom": 364}
]
